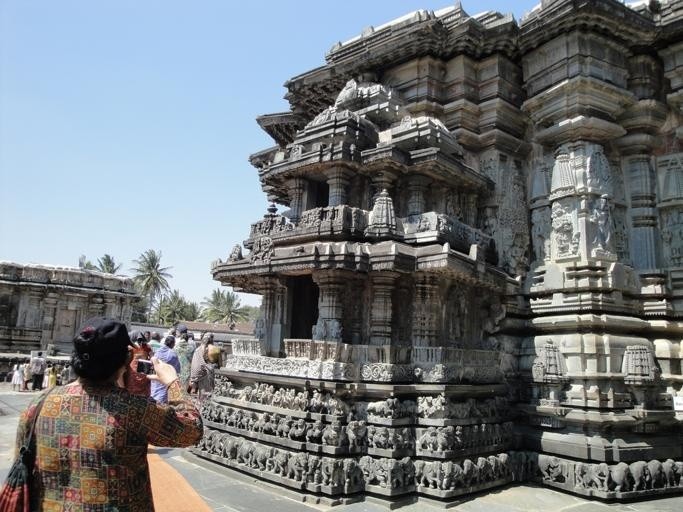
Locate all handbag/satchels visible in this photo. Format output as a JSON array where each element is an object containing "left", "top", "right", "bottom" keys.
[{"left": 0, "top": 386, "right": 57, "bottom": 512}]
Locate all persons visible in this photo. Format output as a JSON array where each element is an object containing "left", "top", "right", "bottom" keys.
[
  {"left": 60, "top": 363, "right": 70, "bottom": 385},
  {"left": 123, "top": 322, "right": 222, "bottom": 405},
  {"left": 29, "top": 351, "right": 46, "bottom": 391},
  {"left": 0, "top": 315, "right": 205, "bottom": 512},
  {"left": 8, "top": 359, "right": 58, "bottom": 392}
]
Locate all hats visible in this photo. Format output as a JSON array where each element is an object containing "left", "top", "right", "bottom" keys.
[{"left": 72, "top": 315, "right": 142, "bottom": 362}]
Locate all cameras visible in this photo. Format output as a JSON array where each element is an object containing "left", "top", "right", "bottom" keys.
[{"left": 136, "top": 359, "right": 156, "bottom": 375}]
